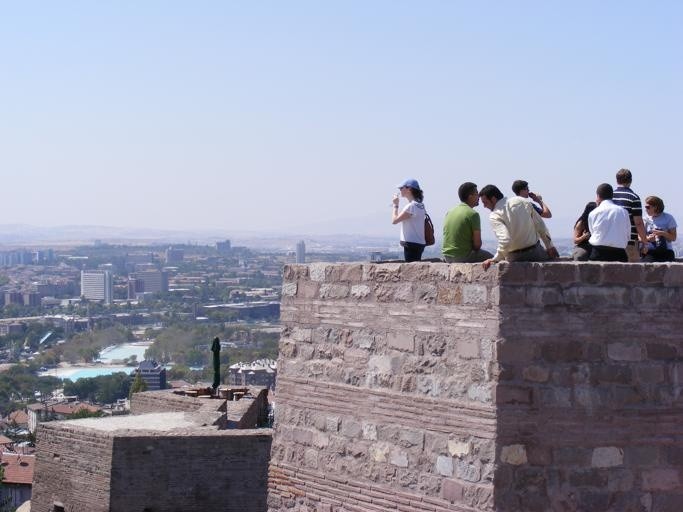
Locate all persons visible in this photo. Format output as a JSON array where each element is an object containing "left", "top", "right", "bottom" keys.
[
  {"left": 638, "top": 196, "right": 677, "bottom": 263},
  {"left": 511, "top": 179, "right": 552, "bottom": 221},
  {"left": 439, "top": 182, "right": 493, "bottom": 262},
  {"left": 476, "top": 184, "right": 560, "bottom": 271},
  {"left": 587, "top": 182, "right": 629, "bottom": 262},
  {"left": 390, "top": 176, "right": 427, "bottom": 262},
  {"left": 569, "top": 201, "right": 596, "bottom": 262},
  {"left": 610, "top": 168, "right": 647, "bottom": 263}
]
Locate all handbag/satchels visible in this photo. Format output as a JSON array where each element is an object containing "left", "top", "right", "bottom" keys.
[{"left": 424, "top": 213, "right": 435, "bottom": 244}]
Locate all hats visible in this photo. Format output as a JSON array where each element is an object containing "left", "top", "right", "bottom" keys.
[{"left": 397, "top": 179, "right": 419, "bottom": 190}]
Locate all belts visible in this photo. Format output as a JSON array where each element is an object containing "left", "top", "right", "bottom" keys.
[
  {"left": 510, "top": 239, "right": 540, "bottom": 254},
  {"left": 628, "top": 240, "right": 635, "bottom": 245}
]
